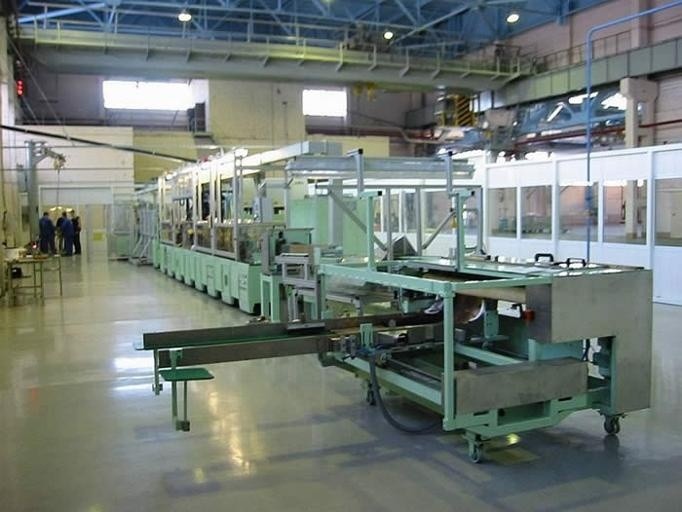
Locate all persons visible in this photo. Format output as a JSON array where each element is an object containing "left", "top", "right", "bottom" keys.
[{"left": 40, "top": 209, "right": 82, "bottom": 257}]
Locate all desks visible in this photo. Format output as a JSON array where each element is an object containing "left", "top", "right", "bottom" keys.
[{"left": 3, "top": 254, "right": 63, "bottom": 308}]
